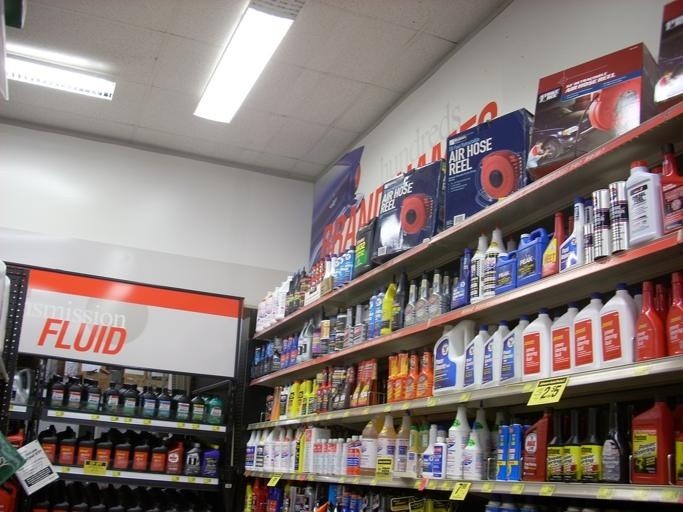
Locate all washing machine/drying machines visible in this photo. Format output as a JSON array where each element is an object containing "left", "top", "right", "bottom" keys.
[{"left": 1, "top": 369, "right": 225, "bottom": 512}]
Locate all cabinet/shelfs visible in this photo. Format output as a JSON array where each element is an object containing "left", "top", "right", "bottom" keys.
[
  {"left": 0, "top": 260, "right": 246, "bottom": 511},
  {"left": 233, "top": 101, "right": 682, "bottom": 511}
]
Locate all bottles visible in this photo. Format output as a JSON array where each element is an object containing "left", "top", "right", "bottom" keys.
[
  {"left": 246, "top": 153, "right": 683, "bottom": 511},
  {"left": 547, "top": 402, "right": 629, "bottom": 483},
  {"left": 404, "top": 347, "right": 431, "bottom": 399},
  {"left": 308, "top": 435, "right": 362, "bottom": 475},
  {"left": 432, "top": 408, "right": 523, "bottom": 481},
  {"left": 254, "top": 245, "right": 354, "bottom": 328},
  {"left": 377, "top": 410, "right": 410, "bottom": 479},
  {"left": 46, "top": 375, "right": 227, "bottom": 425}
]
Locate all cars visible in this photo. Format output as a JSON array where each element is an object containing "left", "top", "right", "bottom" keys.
[{"left": 536, "top": 105, "right": 595, "bottom": 157}]
